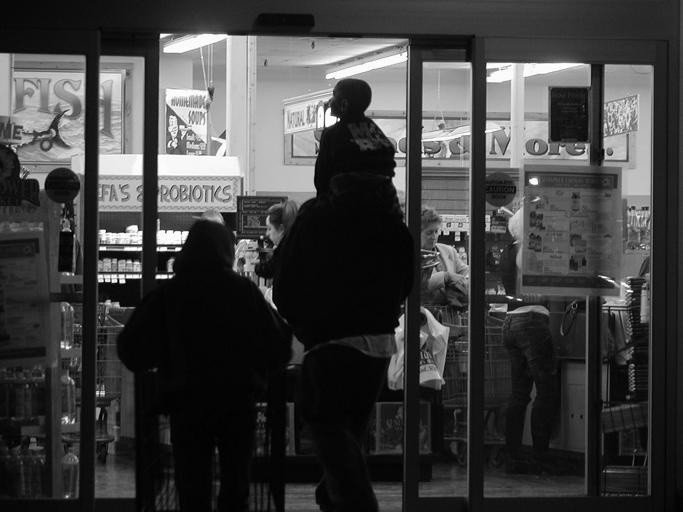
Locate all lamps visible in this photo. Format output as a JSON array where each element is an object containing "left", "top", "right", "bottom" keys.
[
  {"left": 486, "top": 63, "right": 581, "bottom": 84},
  {"left": 162, "top": 33, "right": 226, "bottom": 53},
  {"left": 325, "top": 42, "right": 407, "bottom": 83},
  {"left": 422, "top": 70, "right": 502, "bottom": 142},
  {"left": 604, "top": 93, "right": 638, "bottom": 137}
]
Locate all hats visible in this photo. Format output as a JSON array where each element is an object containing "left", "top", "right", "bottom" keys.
[{"left": 192, "top": 209, "right": 224, "bottom": 224}]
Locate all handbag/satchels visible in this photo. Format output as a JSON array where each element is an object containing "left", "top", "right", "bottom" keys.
[{"left": 441, "top": 283, "right": 469, "bottom": 309}]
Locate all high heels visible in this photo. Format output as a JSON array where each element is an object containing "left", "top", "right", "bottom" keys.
[{"left": 527, "top": 454, "right": 559, "bottom": 476}]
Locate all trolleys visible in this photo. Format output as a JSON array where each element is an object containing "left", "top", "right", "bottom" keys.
[
  {"left": 64, "top": 301, "right": 126, "bottom": 464},
  {"left": 421, "top": 303, "right": 513, "bottom": 470},
  {"left": 147, "top": 388, "right": 275, "bottom": 511}
]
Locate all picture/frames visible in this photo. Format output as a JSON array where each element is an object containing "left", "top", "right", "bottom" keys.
[
  {"left": 9, "top": 69, "right": 126, "bottom": 174},
  {"left": 283, "top": 111, "right": 550, "bottom": 167}
]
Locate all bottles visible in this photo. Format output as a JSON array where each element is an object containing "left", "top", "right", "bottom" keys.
[
  {"left": 58, "top": 301, "right": 79, "bottom": 499},
  {"left": 626, "top": 206, "right": 650, "bottom": 250}
]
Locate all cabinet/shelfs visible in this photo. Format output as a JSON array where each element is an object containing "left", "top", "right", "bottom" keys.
[
  {"left": 0, "top": 201, "right": 77, "bottom": 510},
  {"left": 75, "top": 174, "right": 241, "bottom": 476}
]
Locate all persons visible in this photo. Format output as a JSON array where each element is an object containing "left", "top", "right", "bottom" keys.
[
  {"left": 116, "top": 219, "right": 292, "bottom": 512},
  {"left": 499, "top": 223, "right": 560, "bottom": 474},
  {"left": 206, "top": 79, "right": 407, "bottom": 512},
  {"left": 419, "top": 206, "right": 471, "bottom": 459}
]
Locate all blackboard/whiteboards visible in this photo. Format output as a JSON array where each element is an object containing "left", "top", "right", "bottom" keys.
[{"left": 547, "top": 86, "right": 592, "bottom": 143}]
